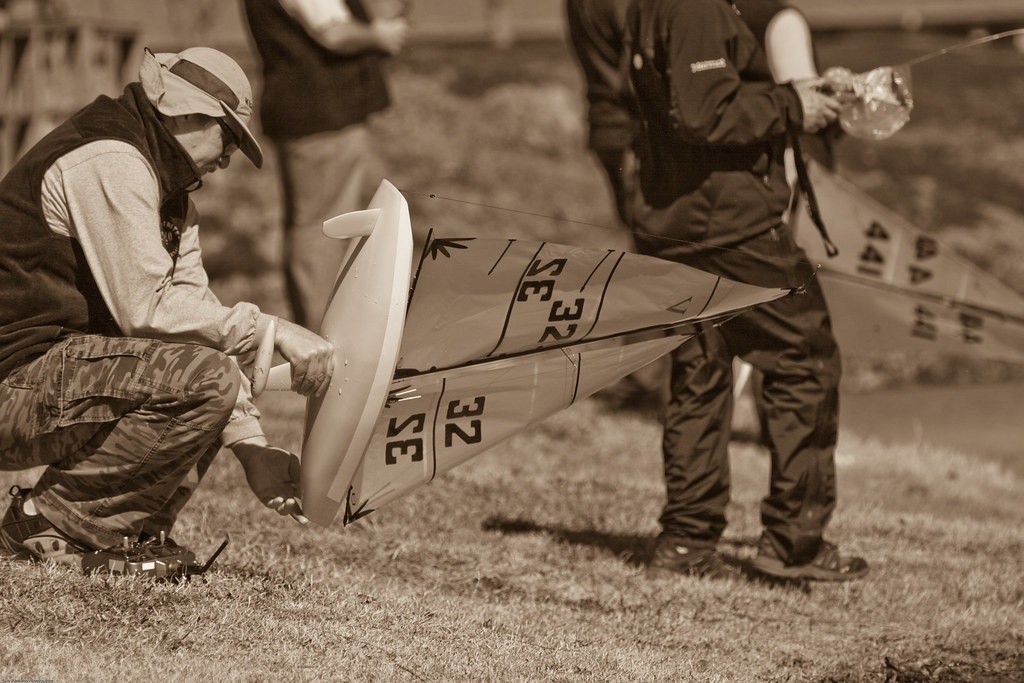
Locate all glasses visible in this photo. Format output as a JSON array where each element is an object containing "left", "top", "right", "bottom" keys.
[{"left": 214, "top": 116, "right": 241, "bottom": 158}]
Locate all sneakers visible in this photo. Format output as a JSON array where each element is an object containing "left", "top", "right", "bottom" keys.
[
  {"left": 648, "top": 539, "right": 744, "bottom": 582},
  {"left": 750, "top": 534, "right": 870, "bottom": 579},
  {"left": 0, "top": 484, "right": 197, "bottom": 576}
]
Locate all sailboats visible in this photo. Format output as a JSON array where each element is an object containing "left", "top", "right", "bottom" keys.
[{"left": 253, "top": 180, "right": 809, "bottom": 517}]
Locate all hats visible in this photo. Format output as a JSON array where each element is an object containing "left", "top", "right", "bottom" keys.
[{"left": 138, "top": 46, "right": 264, "bottom": 169}]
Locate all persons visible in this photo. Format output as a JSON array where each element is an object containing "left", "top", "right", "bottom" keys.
[
  {"left": 243, "top": 0, "right": 408, "bottom": 326},
  {"left": 560, "top": 0, "right": 872, "bottom": 582},
  {"left": 0, "top": 49, "right": 334, "bottom": 578},
  {"left": 725, "top": 2, "right": 824, "bottom": 444}
]
